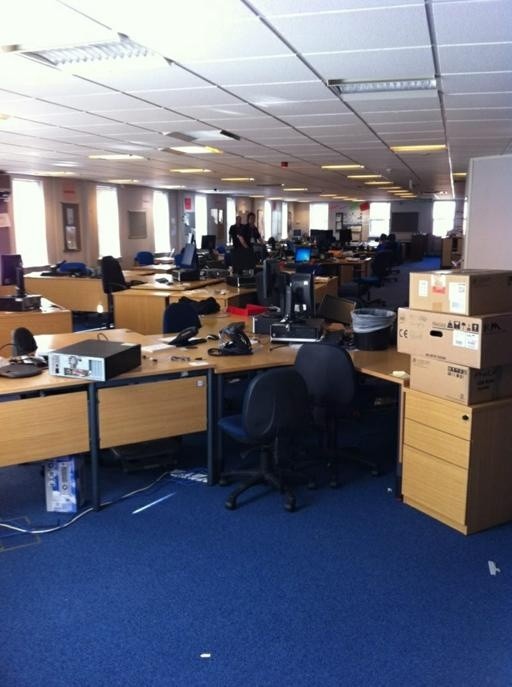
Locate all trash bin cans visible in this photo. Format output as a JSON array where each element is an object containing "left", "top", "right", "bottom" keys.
[{"left": 349, "top": 308, "right": 395, "bottom": 351}]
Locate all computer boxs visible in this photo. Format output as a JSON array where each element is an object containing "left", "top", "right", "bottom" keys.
[
  {"left": 253, "top": 311, "right": 313, "bottom": 334},
  {"left": 270, "top": 318, "right": 325, "bottom": 343},
  {"left": 0, "top": 295, "right": 42, "bottom": 311},
  {"left": 226, "top": 275, "right": 257, "bottom": 288},
  {"left": 49, "top": 339, "right": 142, "bottom": 381},
  {"left": 172, "top": 269, "right": 200, "bottom": 281}
]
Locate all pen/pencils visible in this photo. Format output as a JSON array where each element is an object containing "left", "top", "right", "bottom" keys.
[{"left": 143, "top": 354, "right": 157, "bottom": 362}]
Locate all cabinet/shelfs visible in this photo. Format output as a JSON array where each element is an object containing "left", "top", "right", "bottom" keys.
[
  {"left": 402, "top": 386, "right": 512, "bottom": 538},
  {"left": 440, "top": 235, "right": 463, "bottom": 269}
]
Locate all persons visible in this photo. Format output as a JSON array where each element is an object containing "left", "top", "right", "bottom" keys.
[
  {"left": 238, "top": 212, "right": 263, "bottom": 248},
  {"left": 375, "top": 232, "right": 396, "bottom": 251},
  {"left": 229, "top": 216, "right": 244, "bottom": 246}
]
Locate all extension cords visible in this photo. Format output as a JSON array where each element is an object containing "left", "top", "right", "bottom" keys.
[{"left": 170, "top": 469, "right": 209, "bottom": 483}]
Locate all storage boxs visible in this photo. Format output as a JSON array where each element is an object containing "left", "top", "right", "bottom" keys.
[
  {"left": 395, "top": 305, "right": 511, "bottom": 371},
  {"left": 406, "top": 354, "right": 511, "bottom": 408},
  {"left": 407, "top": 268, "right": 511, "bottom": 318}
]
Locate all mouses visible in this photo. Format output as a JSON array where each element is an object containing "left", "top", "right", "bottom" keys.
[{"left": 167, "top": 282, "right": 173, "bottom": 285}]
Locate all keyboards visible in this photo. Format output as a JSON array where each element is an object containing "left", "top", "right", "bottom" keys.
[{"left": 155, "top": 278, "right": 168, "bottom": 283}]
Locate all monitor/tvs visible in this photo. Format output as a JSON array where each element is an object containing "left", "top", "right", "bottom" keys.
[
  {"left": 293, "top": 228, "right": 353, "bottom": 251},
  {"left": 0, "top": 254, "right": 27, "bottom": 297},
  {"left": 201, "top": 235, "right": 216, "bottom": 254},
  {"left": 280, "top": 273, "right": 316, "bottom": 324},
  {"left": 260, "top": 257, "right": 290, "bottom": 318},
  {"left": 224, "top": 249, "right": 251, "bottom": 276},
  {"left": 187, "top": 233, "right": 196, "bottom": 244},
  {"left": 294, "top": 245, "right": 312, "bottom": 263},
  {"left": 181, "top": 244, "right": 200, "bottom": 271}
]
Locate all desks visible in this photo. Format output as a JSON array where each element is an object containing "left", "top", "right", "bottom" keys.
[{"left": 1, "top": 256, "right": 411, "bottom": 512}]
